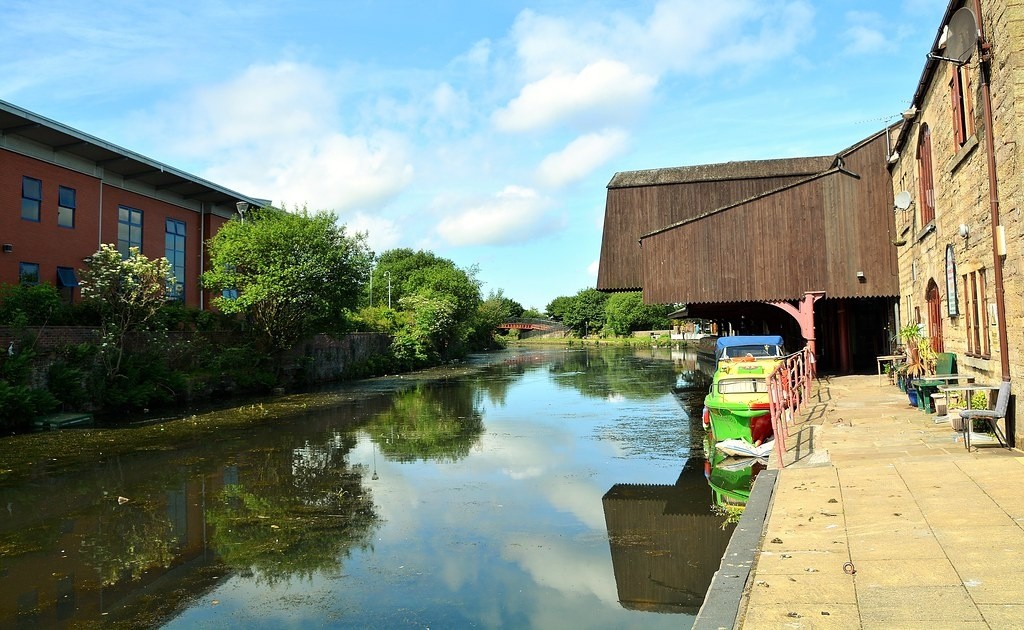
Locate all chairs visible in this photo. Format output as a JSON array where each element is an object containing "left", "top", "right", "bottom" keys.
[{"left": 959, "top": 381, "right": 1011, "bottom": 452}]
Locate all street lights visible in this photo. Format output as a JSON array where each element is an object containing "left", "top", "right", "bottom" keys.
[
  {"left": 237, "top": 202, "right": 249, "bottom": 225},
  {"left": 384, "top": 271, "right": 392, "bottom": 310}
]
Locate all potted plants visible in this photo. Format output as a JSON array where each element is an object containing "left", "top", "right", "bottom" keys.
[{"left": 891, "top": 319, "right": 939, "bottom": 406}]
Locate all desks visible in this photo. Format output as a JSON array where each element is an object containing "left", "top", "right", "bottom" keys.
[
  {"left": 876, "top": 355, "right": 907, "bottom": 387},
  {"left": 937, "top": 383, "right": 1000, "bottom": 442},
  {"left": 924, "top": 374, "right": 975, "bottom": 423}
]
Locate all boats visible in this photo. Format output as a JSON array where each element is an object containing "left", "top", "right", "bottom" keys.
[
  {"left": 712, "top": 336, "right": 788, "bottom": 380},
  {"left": 699, "top": 438, "right": 769, "bottom": 515},
  {"left": 701, "top": 355, "right": 788, "bottom": 447}
]
